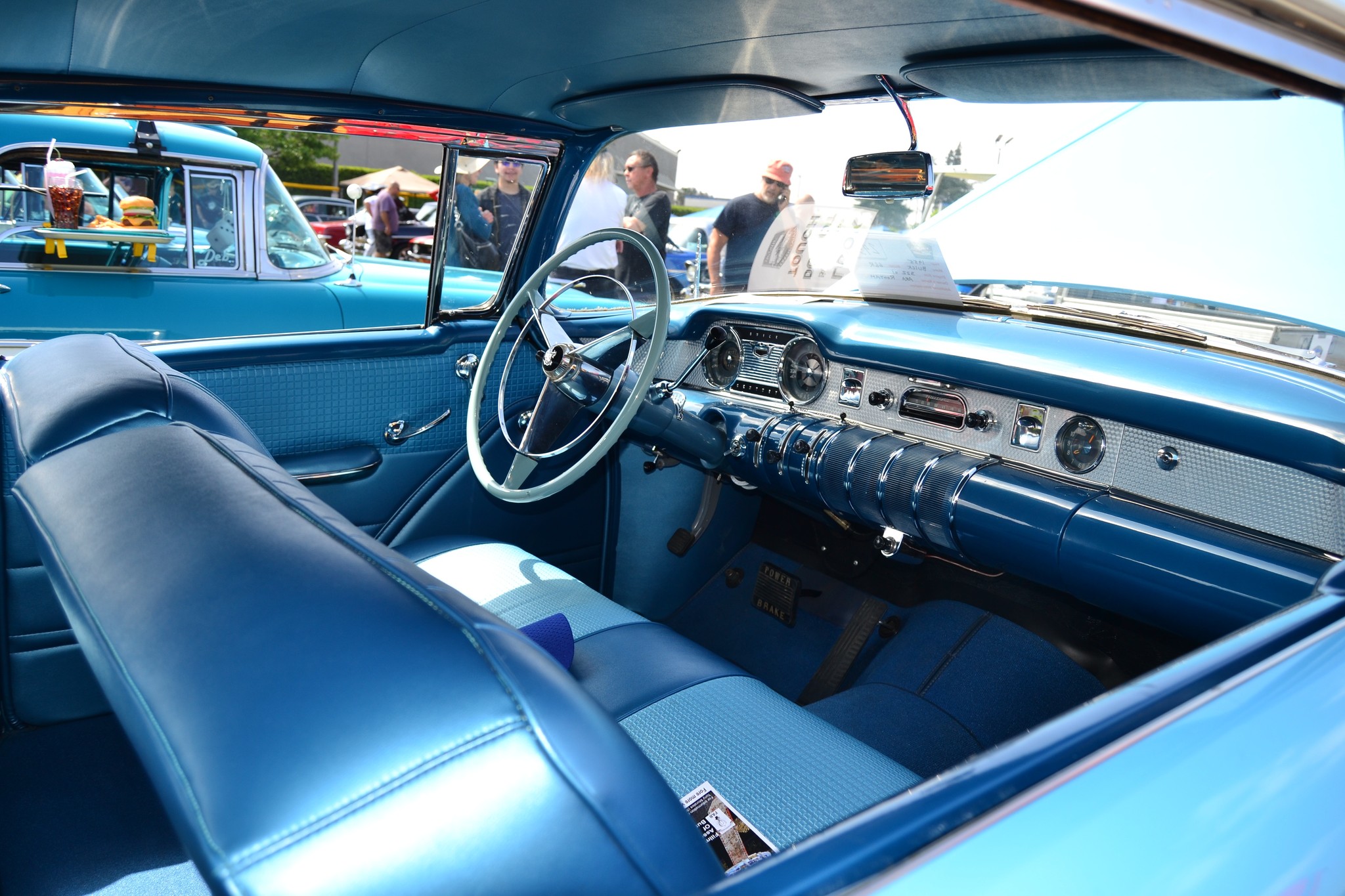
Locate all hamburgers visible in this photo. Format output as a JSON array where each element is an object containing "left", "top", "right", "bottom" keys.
[{"left": 119, "top": 195, "right": 160, "bottom": 229}]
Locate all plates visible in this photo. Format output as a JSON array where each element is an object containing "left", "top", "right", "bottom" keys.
[{"left": 77, "top": 226, "right": 165, "bottom": 233}]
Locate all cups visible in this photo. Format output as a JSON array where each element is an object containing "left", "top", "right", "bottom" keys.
[
  {"left": 48, "top": 177, "right": 83, "bottom": 229},
  {"left": 44, "top": 166, "right": 76, "bottom": 219}
]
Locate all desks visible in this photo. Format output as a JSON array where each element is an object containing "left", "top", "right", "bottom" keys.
[{"left": 32, "top": 224, "right": 175, "bottom": 262}]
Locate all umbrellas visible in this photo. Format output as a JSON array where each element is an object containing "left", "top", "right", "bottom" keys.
[{"left": 340, "top": 166, "right": 439, "bottom": 199}]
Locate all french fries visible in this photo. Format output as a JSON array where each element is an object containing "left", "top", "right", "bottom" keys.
[{"left": 88, "top": 214, "right": 125, "bottom": 228}]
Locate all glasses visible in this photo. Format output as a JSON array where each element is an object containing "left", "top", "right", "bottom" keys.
[
  {"left": 500, "top": 161, "right": 521, "bottom": 168},
  {"left": 622, "top": 165, "right": 635, "bottom": 173},
  {"left": 765, "top": 177, "right": 786, "bottom": 188}
]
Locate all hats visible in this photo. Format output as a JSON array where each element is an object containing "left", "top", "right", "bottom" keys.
[
  {"left": 434, "top": 154, "right": 490, "bottom": 175},
  {"left": 762, "top": 159, "right": 793, "bottom": 185}
]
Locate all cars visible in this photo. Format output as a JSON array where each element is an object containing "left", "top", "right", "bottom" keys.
[
  {"left": 0, "top": 112, "right": 659, "bottom": 361},
  {"left": 290, "top": 194, "right": 365, "bottom": 253},
  {"left": 0, "top": 1, "right": 1344, "bottom": 896}
]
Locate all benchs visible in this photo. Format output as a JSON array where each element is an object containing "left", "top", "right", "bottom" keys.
[{"left": 0, "top": 333, "right": 924, "bottom": 896}]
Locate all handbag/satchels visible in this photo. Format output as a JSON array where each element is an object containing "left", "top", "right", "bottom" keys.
[{"left": 453, "top": 183, "right": 502, "bottom": 271}]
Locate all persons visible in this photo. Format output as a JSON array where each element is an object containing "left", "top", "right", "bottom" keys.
[
  {"left": 705, "top": 161, "right": 793, "bottom": 296},
  {"left": 167, "top": 181, "right": 188, "bottom": 227},
  {"left": 360, "top": 181, "right": 416, "bottom": 258},
  {"left": 550, "top": 150, "right": 627, "bottom": 300},
  {"left": 195, "top": 179, "right": 225, "bottom": 231},
  {"left": 615, "top": 149, "right": 671, "bottom": 305},
  {"left": 478, "top": 158, "right": 532, "bottom": 271},
  {"left": 434, "top": 155, "right": 493, "bottom": 269},
  {"left": 101, "top": 174, "right": 137, "bottom": 203},
  {"left": 797, "top": 193, "right": 816, "bottom": 222}
]
